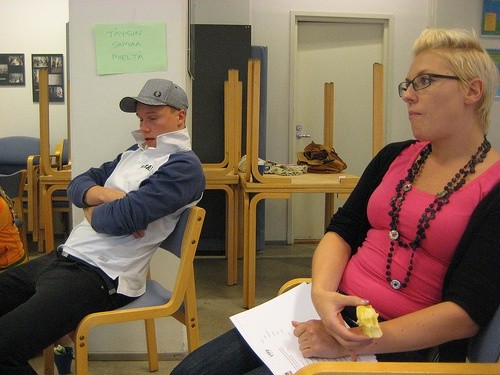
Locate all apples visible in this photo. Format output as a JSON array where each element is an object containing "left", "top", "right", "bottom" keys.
[{"left": 356, "top": 305, "right": 383, "bottom": 338}]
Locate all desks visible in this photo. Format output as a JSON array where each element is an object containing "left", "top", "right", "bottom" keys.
[{"left": 39, "top": 59, "right": 384, "bottom": 307}]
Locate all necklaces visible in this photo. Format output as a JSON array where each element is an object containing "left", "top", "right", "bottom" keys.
[{"left": 386, "top": 136, "right": 492, "bottom": 290}]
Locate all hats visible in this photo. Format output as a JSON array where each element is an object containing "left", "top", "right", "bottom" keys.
[{"left": 120, "top": 78, "right": 189, "bottom": 113}]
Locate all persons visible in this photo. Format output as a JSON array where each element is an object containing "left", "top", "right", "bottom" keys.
[
  {"left": 0, "top": 185, "right": 29, "bottom": 273},
  {"left": 170, "top": 29, "right": 500, "bottom": 375},
  {"left": 0, "top": 78, "right": 205, "bottom": 375}
]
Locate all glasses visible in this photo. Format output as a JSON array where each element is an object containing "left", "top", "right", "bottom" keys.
[{"left": 398, "top": 73, "right": 459, "bottom": 97}]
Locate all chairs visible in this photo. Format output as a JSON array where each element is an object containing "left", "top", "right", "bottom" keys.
[
  {"left": 277, "top": 279, "right": 500, "bottom": 375},
  {"left": 0, "top": 136, "right": 206, "bottom": 375}
]
